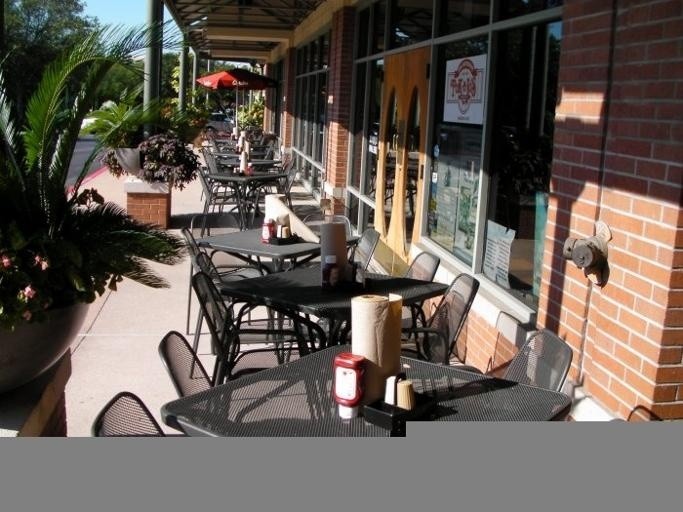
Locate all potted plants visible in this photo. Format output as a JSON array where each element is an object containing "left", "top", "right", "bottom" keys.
[
  {"left": 79, "top": 81, "right": 212, "bottom": 175},
  {"left": 1, "top": 18, "right": 199, "bottom": 392}
]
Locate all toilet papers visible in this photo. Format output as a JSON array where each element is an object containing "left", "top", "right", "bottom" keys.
[
  {"left": 240, "top": 152, "right": 248, "bottom": 173},
  {"left": 233, "top": 127, "right": 238, "bottom": 138},
  {"left": 265, "top": 194, "right": 321, "bottom": 243},
  {"left": 244, "top": 141, "right": 251, "bottom": 161},
  {"left": 349, "top": 293, "right": 405, "bottom": 403},
  {"left": 240, "top": 131, "right": 248, "bottom": 148},
  {"left": 320, "top": 222, "right": 352, "bottom": 283}
]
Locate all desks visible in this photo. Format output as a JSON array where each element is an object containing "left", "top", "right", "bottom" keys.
[
  {"left": 200, "top": 139, "right": 294, "bottom": 238},
  {"left": 192, "top": 227, "right": 361, "bottom": 330},
  {"left": 162, "top": 344, "right": 571, "bottom": 437},
  {"left": 214, "top": 262, "right": 450, "bottom": 419}
]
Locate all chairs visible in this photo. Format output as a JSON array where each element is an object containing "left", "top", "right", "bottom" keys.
[
  {"left": 502, "top": 329, "right": 574, "bottom": 393},
  {"left": 192, "top": 271, "right": 323, "bottom": 424},
  {"left": 159, "top": 331, "right": 213, "bottom": 398},
  {"left": 198, "top": 132, "right": 243, "bottom": 239},
  {"left": 189, "top": 252, "right": 297, "bottom": 381},
  {"left": 401, "top": 274, "right": 479, "bottom": 398},
  {"left": 353, "top": 229, "right": 380, "bottom": 272},
  {"left": 251, "top": 132, "right": 297, "bottom": 224},
  {"left": 91, "top": 391, "right": 166, "bottom": 436},
  {"left": 401, "top": 251, "right": 440, "bottom": 353},
  {"left": 180, "top": 226, "right": 252, "bottom": 325}
]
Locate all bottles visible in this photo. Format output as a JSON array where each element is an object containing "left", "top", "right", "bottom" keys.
[
  {"left": 355, "top": 268, "right": 365, "bottom": 288},
  {"left": 322, "top": 255, "right": 340, "bottom": 294},
  {"left": 396, "top": 379, "right": 414, "bottom": 412},
  {"left": 261, "top": 218, "right": 273, "bottom": 242},
  {"left": 333, "top": 354, "right": 363, "bottom": 420},
  {"left": 282, "top": 227, "right": 288, "bottom": 239},
  {"left": 245, "top": 163, "right": 254, "bottom": 174},
  {"left": 384, "top": 375, "right": 397, "bottom": 407},
  {"left": 276, "top": 225, "right": 281, "bottom": 238}
]
